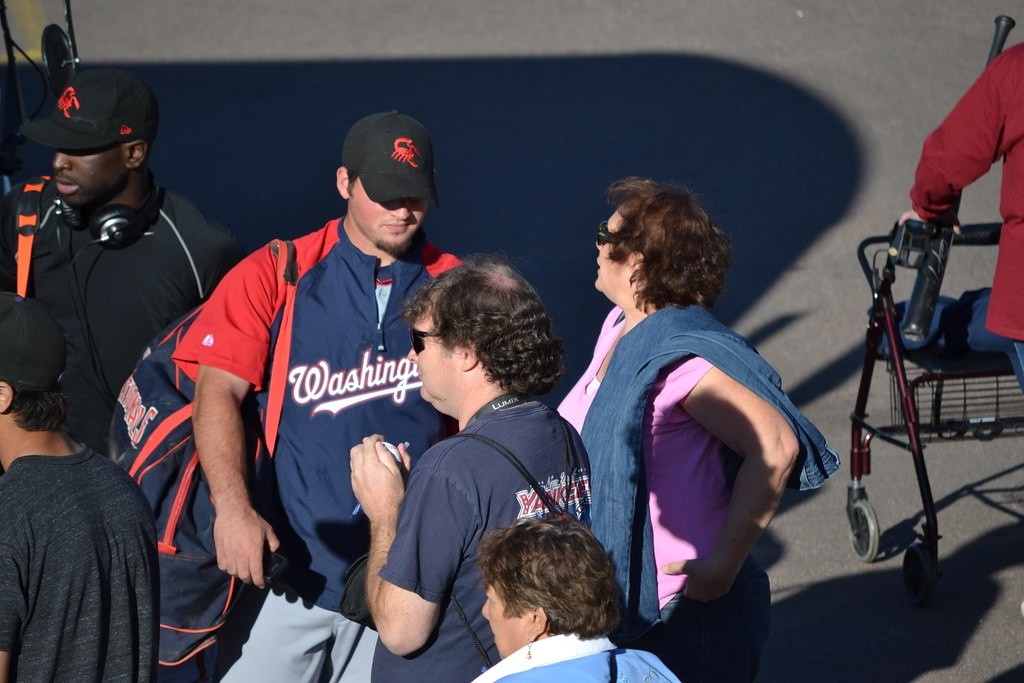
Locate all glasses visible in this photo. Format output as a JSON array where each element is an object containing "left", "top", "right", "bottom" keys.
[
  {"left": 409, "top": 327, "right": 442, "bottom": 355},
  {"left": 596, "top": 222, "right": 624, "bottom": 246}
]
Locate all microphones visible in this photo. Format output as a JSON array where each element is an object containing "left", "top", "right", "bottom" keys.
[{"left": 36, "top": 206, "right": 55, "bottom": 233}]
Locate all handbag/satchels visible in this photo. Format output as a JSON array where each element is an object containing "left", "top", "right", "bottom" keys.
[
  {"left": 108, "top": 239, "right": 299, "bottom": 665},
  {"left": 340, "top": 554, "right": 383, "bottom": 632}
]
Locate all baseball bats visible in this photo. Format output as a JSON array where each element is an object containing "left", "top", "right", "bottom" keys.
[{"left": 895, "top": 14, "right": 1016, "bottom": 345}]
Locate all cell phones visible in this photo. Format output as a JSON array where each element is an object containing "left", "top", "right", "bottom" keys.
[{"left": 262, "top": 548, "right": 288, "bottom": 584}]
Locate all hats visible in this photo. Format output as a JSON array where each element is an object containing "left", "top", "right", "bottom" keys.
[
  {"left": 0, "top": 292, "right": 67, "bottom": 392},
  {"left": 20, "top": 68, "right": 158, "bottom": 149},
  {"left": 342, "top": 110, "right": 440, "bottom": 209}
]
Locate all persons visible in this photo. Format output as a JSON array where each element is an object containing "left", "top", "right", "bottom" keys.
[
  {"left": 191, "top": 111, "right": 468, "bottom": 681},
  {"left": 554, "top": 174, "right": 801, "bottom": 683},
  {"left": 899, "top": 41, "right": 1024, "bottom": 392},
  {"left": 348, "top": 257, "right": 595, "bottom": 683},
  {"left": 0, "top": 283, "right": 165, "bottom": 681},
  {"left": 0, "top": 66, "right": 247, "bottom": 459},
  {"left": 475, "top": 522, "right": 681, "bottom": 683}
]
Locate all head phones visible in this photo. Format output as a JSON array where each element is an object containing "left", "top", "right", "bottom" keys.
[{"left": 52, "top": 181, "right": 160, "bottom": 249}]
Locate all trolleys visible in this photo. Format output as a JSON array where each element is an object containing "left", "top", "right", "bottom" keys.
[{"left": 844, "top": 217, "right": 1024, "bottom": 604}]
